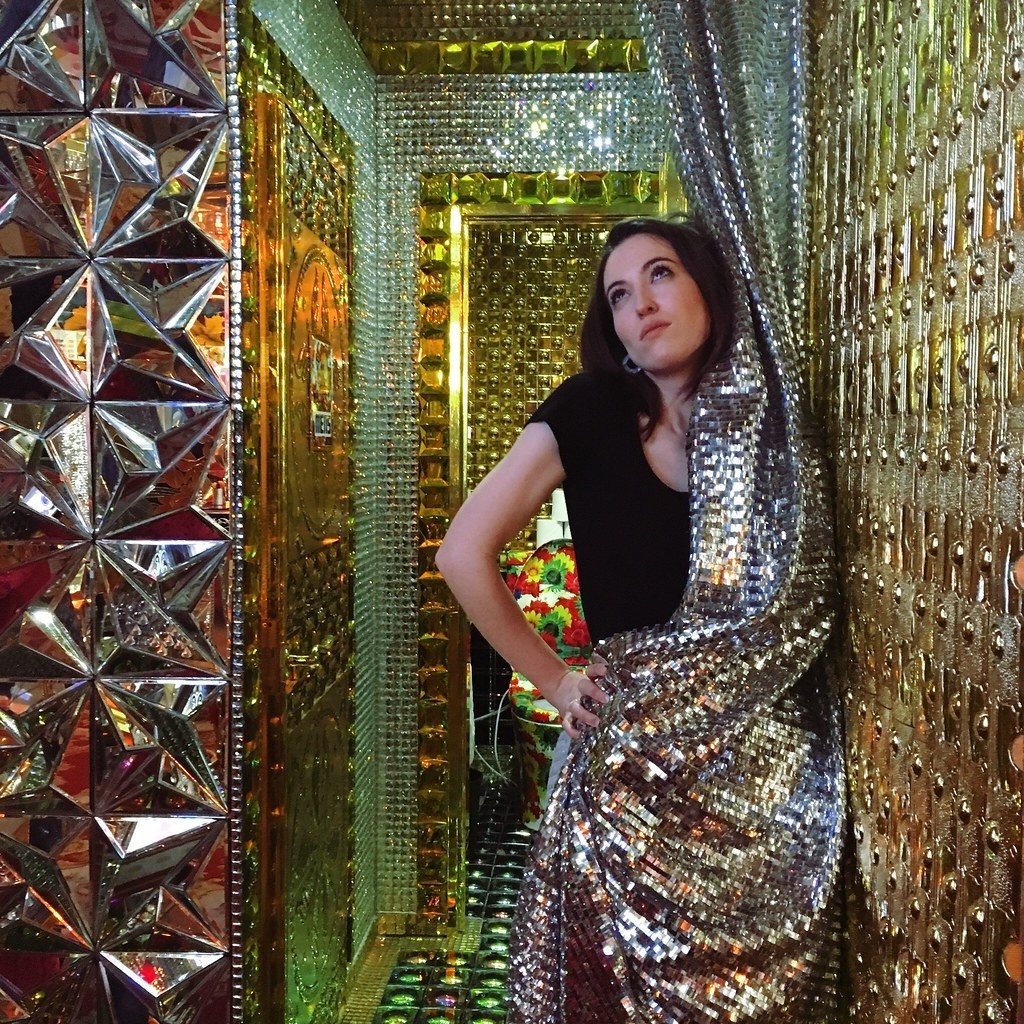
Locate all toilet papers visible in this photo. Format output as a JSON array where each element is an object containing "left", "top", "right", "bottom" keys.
[{"left": 536, "top": 489, "right": 572, "bottom": 548}]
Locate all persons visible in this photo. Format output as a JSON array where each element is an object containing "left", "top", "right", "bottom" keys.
[{"left": 435, "top": 220, "right": 737, "bottom": 801}]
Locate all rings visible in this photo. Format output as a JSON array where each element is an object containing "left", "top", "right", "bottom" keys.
[{"left": 569, "top": 698, "right": 579, "bottom": 706}]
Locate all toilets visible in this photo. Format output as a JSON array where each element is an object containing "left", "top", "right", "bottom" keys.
[{"left": 509, "top": 539, "right": 592, "bottom": 831}]
